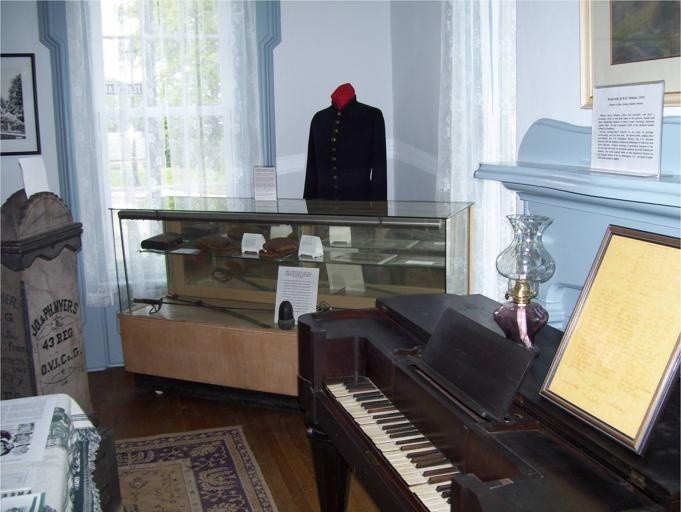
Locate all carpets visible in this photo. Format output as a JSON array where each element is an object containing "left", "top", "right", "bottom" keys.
[{"left": 115, "top": 425, "right": 280, "bottom": 512}]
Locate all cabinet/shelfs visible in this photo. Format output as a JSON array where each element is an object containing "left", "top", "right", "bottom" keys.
[{"left": 109, "top": 196, "right": 477, "bottom": 413}]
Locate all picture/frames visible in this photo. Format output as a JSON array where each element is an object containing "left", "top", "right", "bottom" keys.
[
  {"left": 537, "top": 224, "right": 681, "bottom": 459},
  {"left": 1, "top": 52, "right": 44, "bottom": 158}
]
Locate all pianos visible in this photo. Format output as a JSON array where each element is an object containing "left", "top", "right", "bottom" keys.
[{"left": 298, "top": 294, "right": 681, "bottom": 511}]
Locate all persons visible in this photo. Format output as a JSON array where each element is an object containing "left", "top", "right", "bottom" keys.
[{"left": 301, "top": 83, "right": 389, "bottom": 203}]
